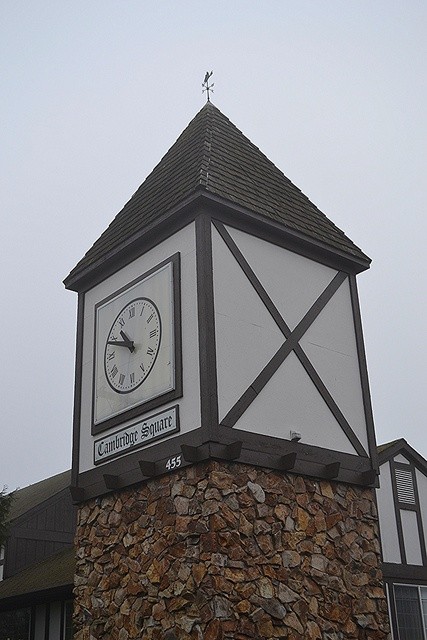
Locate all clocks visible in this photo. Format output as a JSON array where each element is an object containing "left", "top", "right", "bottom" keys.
[{"left": 91, "top": 250, "right": 182, "bottom": 437}]
